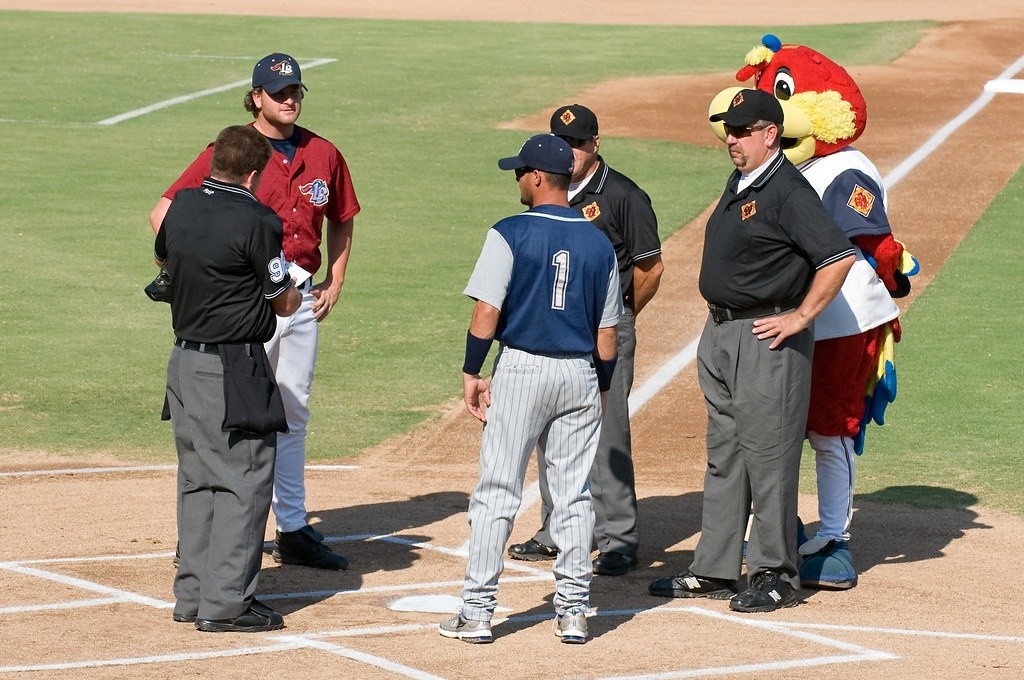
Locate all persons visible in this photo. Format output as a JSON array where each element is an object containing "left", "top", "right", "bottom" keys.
[
  {"left": 438, "top": 136, "right": 624, "bottom": 644},
  {"left": 155, "top": 125, "right": 302, "bottom": 633},
  {"left": 148, "top": 54, "right": 362, "bottom": 570},
  {"left": 709, "top": 34, "right": 919, "bottom": 589},
  {"left": 649, "top": 89, "right": 854, "bottom": 613},
  {"left": 507, "top": 105, "right": 663, "bottom": 578}
]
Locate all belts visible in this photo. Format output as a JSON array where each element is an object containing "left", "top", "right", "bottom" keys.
[
  {"left": 705, "top": 300, "right": 793, "bottom": 324},
  {"left": 167, "top": 334, "right": 223, "bottom": 356}
]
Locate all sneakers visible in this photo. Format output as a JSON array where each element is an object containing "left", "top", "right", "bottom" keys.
[
  {"left": 729, "top": 571, "right": 802, "bottom": 614},
  {"left": 509, "top": 537, "right": 559, "bottom": 561},
  {"left": 648, "top": 569, "right": 742, "bottom": 598},
  {"left": 272, "top": 527, "right": 351, "bottom": 572},
  {"left": 594, "top": 550, "right": 641, "bottom": 578},
  {"left": 194, "top": 605, "right": 284, "bottom": 631},
  {"left": 434, "top": 611, "right": 496, "bottom": 645},
  {"left": 549, "top": 607, "right": 591, "bottom": 647}
]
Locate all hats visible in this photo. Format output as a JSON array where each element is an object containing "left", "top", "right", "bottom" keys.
[
  {"left": 495, "top": 132, "right": 578, "bottom": 175},
  {"left": 549, "top": 103, "right": 601, "bottom": 142},
  {"left": 252, "top": 52, "right": 309, "bottom": 92},
  {"left": 709, "top": 87, "right": 789, "bottom": 129}
]
